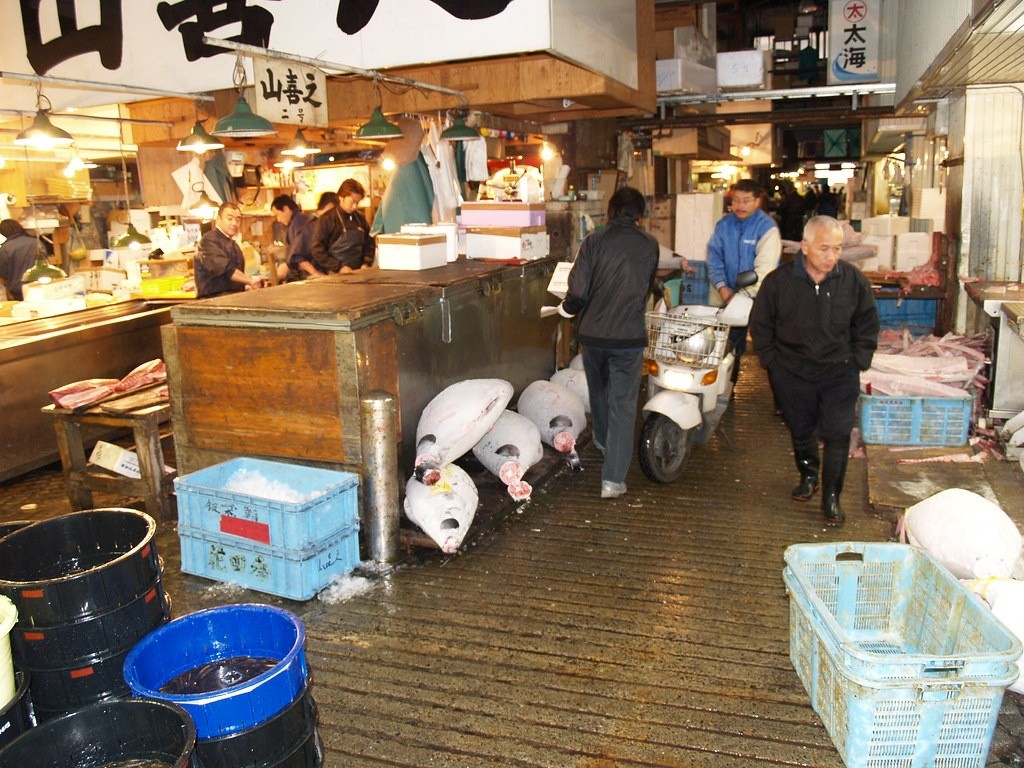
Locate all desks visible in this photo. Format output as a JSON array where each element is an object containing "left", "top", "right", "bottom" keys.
[{"left": 42, "top": 383, "right": 175, "bottom": 523}]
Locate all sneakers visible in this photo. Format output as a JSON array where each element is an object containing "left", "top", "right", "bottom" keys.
[
  {"left": 592, "top": 429, "right": 607, "bottom": 456},
  {"left": 599, "top": 479, "right": 627, "bottom": 498}
]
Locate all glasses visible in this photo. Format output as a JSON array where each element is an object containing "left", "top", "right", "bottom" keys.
[{"left": 731, "top": 197, "right": 756, "bottom": 206}]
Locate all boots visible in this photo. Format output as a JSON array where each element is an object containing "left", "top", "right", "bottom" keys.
[
  {"left": 821, "top": 447, "right": 846, "bottom": 522},
  {"left": 789, "top": 430, "right": 820, "bottom": 501}
]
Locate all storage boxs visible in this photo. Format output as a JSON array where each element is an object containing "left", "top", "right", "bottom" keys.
[
  {"left": 375, "top": 198, "right": 551, "bottom": 271},
  {"left": 681, "top": 260, "right": 710, "bottom": 305},
  {"left": 857, "top": 213, "right": 933, "bottom": 272},
  {"left": 677, "top": 190, "right": 726, "bottom": 260},
  {"left": 653, "top": 60, "right": 716, "bottom": 97},
  {"left": 172, "top": 456, "right": 361, "bottom": 602},
  {"left": 652, "top": 124, "right": 731, "bottom": 162},
  {"left": 714, "top": 51, "right": 769, "bottom": 94}
]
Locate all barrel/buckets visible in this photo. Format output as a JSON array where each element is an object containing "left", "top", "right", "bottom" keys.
[
  {"left": 121, "top": 603, "right": 326, "bottom": 768},
  {"left": 0, "top": 697, "right": 208, "bottom": 768},
  {"left": 0, "top": 594, "right": 41, "bottom": 753},
  {"left": 0, "top": 508, "right": 172, "bottom": 724}
]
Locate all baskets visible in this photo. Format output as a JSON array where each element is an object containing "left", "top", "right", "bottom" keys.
[{"left": 643, "top": 309, "right": 731, "bottom": 369}]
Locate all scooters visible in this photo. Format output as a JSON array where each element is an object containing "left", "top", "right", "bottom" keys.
[{"left": 637, "top": 270, "right": 759, "bottom": 485}]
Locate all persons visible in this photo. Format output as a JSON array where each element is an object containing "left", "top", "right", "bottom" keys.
[
  {"left": 658, "top": 244, "right": 696, "bottom": 272},
  {"left": 749, "top": 216, "right": 880, "bottom": 526},
  {"left": 195, "top": 202, "right": 264, "bottom": 299},
  {"left": 287, "top": 192, "right": 339, "bottom": 276},
  {"left": 258, "top": 195, "right": 309, "bottom": 281},
  {"left": 1, "top": 220, "right": 48, "bottom": 302},
  {"left": 706, "top": 179, "right": 783, "bottom": 415},
  {"left": 777, "top": 185, "right": 847, "bottom": 242},
  {"left": 556, "top": 185, "right": 660, "bottom": 498},
  {"left": 311, "top": 178, "right": 375, "bottom": 273}
]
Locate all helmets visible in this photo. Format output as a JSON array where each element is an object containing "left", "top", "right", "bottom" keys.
[{"left": 673, "top": 324, "right": 714, "bottom": 364}]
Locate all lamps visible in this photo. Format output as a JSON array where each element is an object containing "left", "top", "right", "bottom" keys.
[
  {"left": 176, "top": 101, "right": 227, "bottom": 154},
  {"left": 189, "top": 188, "right": 219, "bottom": 216},
  {"left": 439, "top": 96, "right": 480, "bottom": 143},
  {"left": 110, "top": 224, "right": 152, "bottom": 251},
  {"left": 351, "top": 83, "right": 405, "bottom": 141},
  {"left": 273, "top": 124, "right": 323, "bottom": 171},
  {"left": 209, "top": 52, "right": 278, "bottom": 138},
  {"left": 13, "top": 83, "right": 75, "bottom": 149},
  {"left": 20, "top": 252, "right": 68, "bottom": 284}
]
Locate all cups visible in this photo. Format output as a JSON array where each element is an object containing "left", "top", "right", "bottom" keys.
[
  {"left": 549, "top": 178, "right": 568, "bottom": 201},
  {"left": 494, "top": 189, "right": 502, "bottom": 202}
]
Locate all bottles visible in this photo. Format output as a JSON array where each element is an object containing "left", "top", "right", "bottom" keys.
[
  {"left": 568, "top": 185, "right": 575, "bottom": 201},
  {"left": 549, "top": 191, "right": 553, "bottom": 201},
  {"left": 249, "top": 235, "right": 263, "bottom": 265},
  {"left": 592, "top": 176, "right": 596, "bottom": 191}
]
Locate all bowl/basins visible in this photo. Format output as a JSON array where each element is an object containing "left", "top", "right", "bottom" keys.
[{"left": 578, "top": 190, "right": 606, "bottom": 200}]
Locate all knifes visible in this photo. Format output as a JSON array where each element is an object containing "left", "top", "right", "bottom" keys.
[{"left": 870, "top": 283, "right": 902, "bottom": 288}]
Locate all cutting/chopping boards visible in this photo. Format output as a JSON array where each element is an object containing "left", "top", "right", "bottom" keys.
[{"left": 864, "top": 276, "right": 909, "bottom": 288}]
